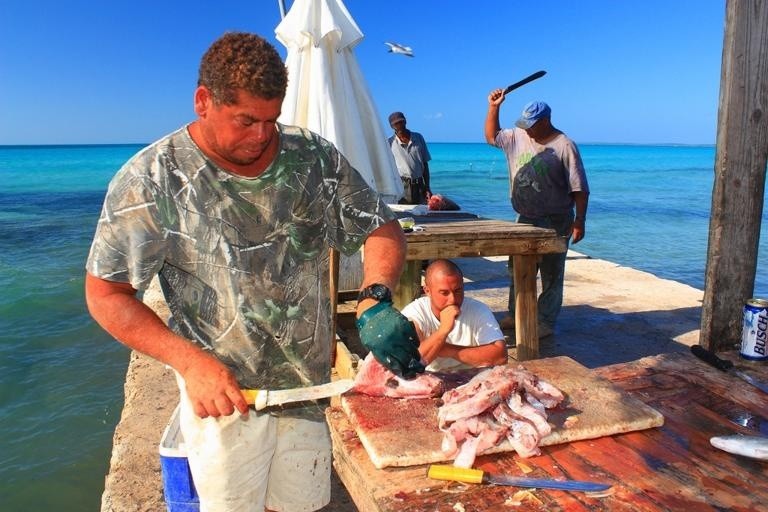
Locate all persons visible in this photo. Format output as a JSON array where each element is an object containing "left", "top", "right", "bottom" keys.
[
  {"left": 84, "top": 33, "right": 423, "bottom": 512},
  {"left": 400, "top": 260, "right": 508, "bottom": 372},
  {"left": 387, "top": 112, "right": 433, "bottom": 204},
  {"left": 484, "top": 90, "right": 589, "bottom": 342}
]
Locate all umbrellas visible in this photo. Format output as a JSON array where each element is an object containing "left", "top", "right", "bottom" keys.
[{"left": 275, "top": 0, "right": 404, "bottom": 368}]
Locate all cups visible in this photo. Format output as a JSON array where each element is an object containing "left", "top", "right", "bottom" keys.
[{"left": 397, "top": 217, "right": 414, "bottom": 232}]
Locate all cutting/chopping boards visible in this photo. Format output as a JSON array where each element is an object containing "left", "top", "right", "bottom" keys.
[
  {"left": 417, "top": 213, "right": 478, "bottom": 217},
  {"left": 341, "top": 354, "right": 665, "bottom": 468}
]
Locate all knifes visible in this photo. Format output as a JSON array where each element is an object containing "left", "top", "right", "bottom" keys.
[
  {"left": 234, "top": 378, "right": 355, "bottom": 412},
  {"left": 691, "top": 345, "right": 768, "bottom": 392},
  {"left": 428, "top": 463, "right": 611, "bottom": 490},
  {"left": 505, "top": 71, "right": 547, "bottom": 94}
]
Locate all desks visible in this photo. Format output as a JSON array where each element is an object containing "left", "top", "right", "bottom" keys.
[
  {"left": 327, "top": 208, "right": 566, "bottom": 362},
  {"left": 320, "top": 349, "right": 767, "bottom": 512}
]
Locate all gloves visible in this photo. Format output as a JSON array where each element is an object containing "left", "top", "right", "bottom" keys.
[{"left": 356, "top": 302, "right": 425, "bottom": 379}]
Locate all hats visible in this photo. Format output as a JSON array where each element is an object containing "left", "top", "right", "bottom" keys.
[
  {"left": 389, "top": 112, "right": 405, "bottom": 125},
  {"left": 515, "top": 101, "right": 551, "bottom": 130}
]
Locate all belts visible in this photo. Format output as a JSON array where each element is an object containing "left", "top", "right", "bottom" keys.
[{"left": 401, "top": 176, "right": 422, "bottom": 184}]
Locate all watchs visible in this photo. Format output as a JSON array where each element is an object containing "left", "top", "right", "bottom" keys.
[{"left": 356, "top": 284, "right": 391, "bottom": 304}]
